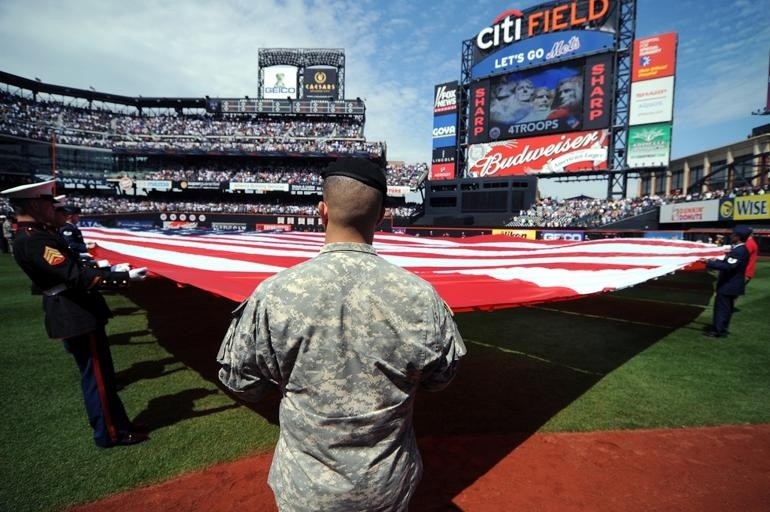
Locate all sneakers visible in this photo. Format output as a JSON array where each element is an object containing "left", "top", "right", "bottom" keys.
[{"left": 703, "top": 326, "right": 727, "bottom": 338}]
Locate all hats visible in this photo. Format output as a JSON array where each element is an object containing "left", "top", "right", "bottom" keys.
[
  {"left": 733, "top": 225, "right": 753, "bottom": 233},
  {"left": 52, "top": 194, "right": 72, "bottom": 212},
  {"left": 1, "top": 179, "right": 61, "bottom": 203},
  {"left": 64, "top": 206, "right": 82, "bottom": 215},
  {"left": 320, "top": 157, "right": 388, "bottom": 194}
]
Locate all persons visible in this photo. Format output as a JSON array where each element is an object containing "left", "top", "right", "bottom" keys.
[
  {"left": 217, "top": 154, "right": 469, "bottom": 511},
  {"left": 1, "top": 177, "right": 150, "bottom": 448},
  {"left": 698, "top": 223, "right": 752, "bottom": 339},
  {"left": 743, "top": 236, "right": 758, "bottom": 285}
]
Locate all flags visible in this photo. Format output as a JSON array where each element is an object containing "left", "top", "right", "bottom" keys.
[{"left": 76, "top": 225, "right": 735, "bottom": 314}]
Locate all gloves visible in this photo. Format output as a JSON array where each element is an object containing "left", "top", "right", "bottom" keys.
[
  {"left": 111, "top": 263, "right": 131, "bottom": 273},
  {"left": 97, "top": 259, "right": 111, "bottom": 268},
  {"left": 128, "top": 267, "right": 147, "bottom": 280}
]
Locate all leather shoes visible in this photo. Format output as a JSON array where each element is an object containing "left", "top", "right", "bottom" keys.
[{"left": 113, "top": 420, "right": 149, "bottom": 446}]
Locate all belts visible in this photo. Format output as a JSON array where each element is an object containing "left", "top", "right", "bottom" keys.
[{"left": 43, "top": 282, "right": 71, "bottom": 296}]
